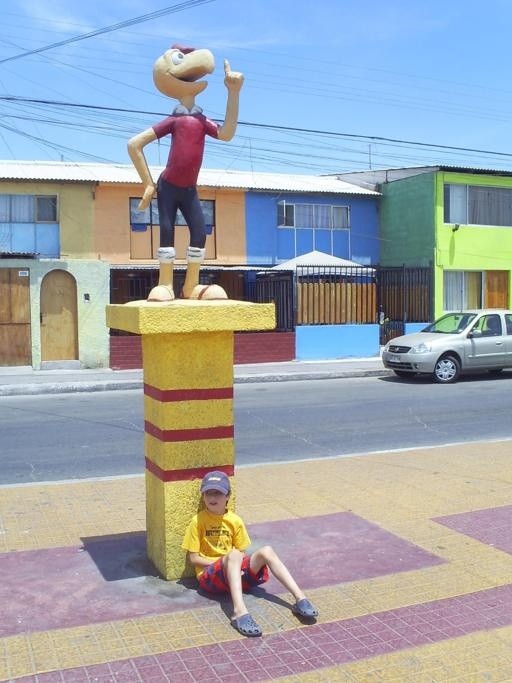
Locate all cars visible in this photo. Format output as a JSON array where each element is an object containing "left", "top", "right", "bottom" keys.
[{"left": 381, "top": 305, "right": 511, "bottom": 382}]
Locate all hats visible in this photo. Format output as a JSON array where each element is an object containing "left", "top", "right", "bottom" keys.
[{"left": 201, "top": 471, "right": 231, "bottom": 494}]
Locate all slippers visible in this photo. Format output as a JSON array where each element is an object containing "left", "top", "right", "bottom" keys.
[
  {"left": 292, "top": 598, "right": 318, "bottom": 617},
  {"left": 231, "top": 613, "right": 262, "bottom": 636}
]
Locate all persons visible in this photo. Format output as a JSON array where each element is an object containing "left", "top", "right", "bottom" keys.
[{"left": 180, "top": 470, "right": 320, "bottom": 639}]
